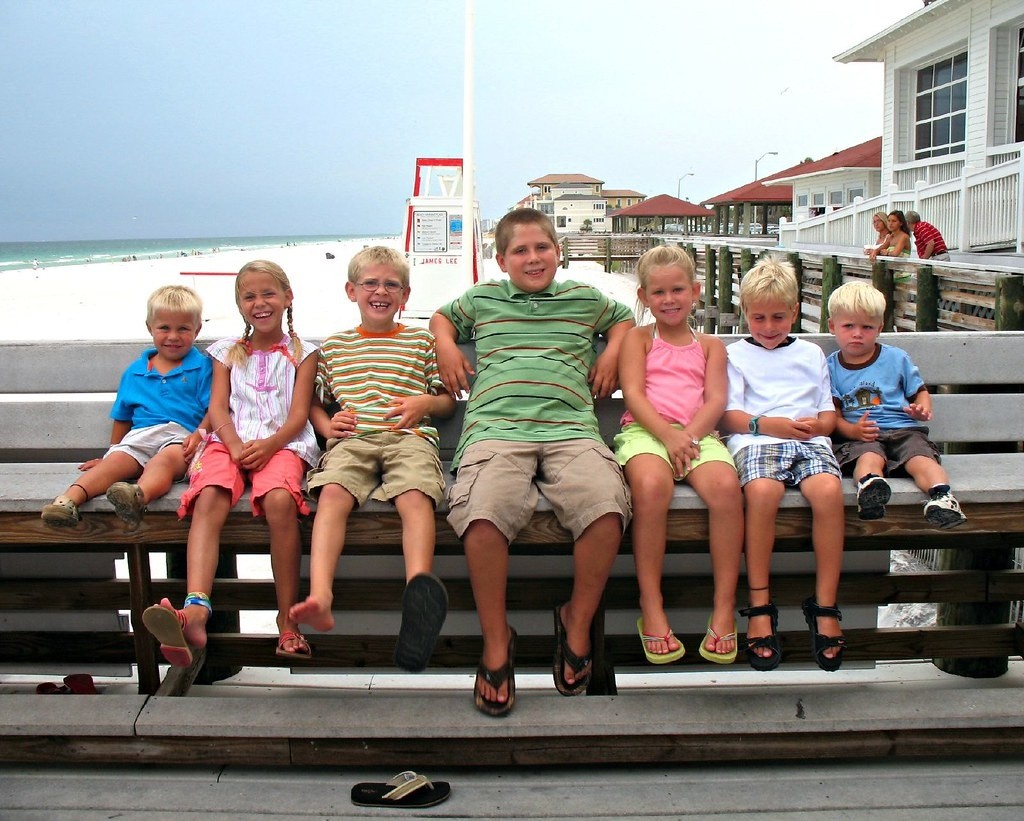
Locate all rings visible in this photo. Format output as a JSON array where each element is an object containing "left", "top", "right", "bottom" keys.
[{"left": 692, "top": 440, "right": 698, "bottom": 446}]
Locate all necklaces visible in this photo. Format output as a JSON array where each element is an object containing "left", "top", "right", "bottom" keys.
[
  {"left": 880, "top": 232, "right": 889, "bottom": 239},
  {"left": 891, "top": 233, "right": 894, "bottom": 238}
]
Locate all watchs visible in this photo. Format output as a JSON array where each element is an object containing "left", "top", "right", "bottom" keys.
[{"left": 748, "top": 415, "right": 767, "bottom": 436}]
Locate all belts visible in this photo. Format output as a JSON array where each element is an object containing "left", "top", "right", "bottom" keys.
[{"left": 932, "top": 250, "right": 949, "bottom": 256}]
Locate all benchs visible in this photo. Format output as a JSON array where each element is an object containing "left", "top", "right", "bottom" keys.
[{"left": 0, "top": 330, "right": 1024, "bottom": 775}]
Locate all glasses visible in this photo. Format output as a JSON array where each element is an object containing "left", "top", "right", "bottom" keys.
[{"left": 358, "top": 280, "right": 408, "bottom": 294}]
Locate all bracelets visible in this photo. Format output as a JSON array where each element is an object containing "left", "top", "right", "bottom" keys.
[
  {"left": 887, "top": 252, "right": 889, "bottom": 255},
  {"left": 216, "top": 422, "right": 232, "bottom": 432}
]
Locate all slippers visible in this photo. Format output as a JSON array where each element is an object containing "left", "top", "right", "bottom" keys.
[
  {"left": 474, "top": 624, "right": 515, "bottom": 717},
  {"left": 637, "top": 617, "right": 686, "bottom": 665},
  {"left": 699, "top": 612, "right": 738, "bottom": 663},
  {"left": 350, "top": 770, "right": 452, "bottom": 808},
  {"left": 276, "top": 634, "right": 311, "bottom": 660},
  {"left": 553, "top": 604, "right": 594, "bottom": 697},
  {"left": 142, "top": 603, "right": 193, "bottom": 667},
  {"left": 394, "top": 574, "right": 452, "bottom": 673},
  {"left": 106, "top": 484, "right": 148, "bottom": 526},
  {"left": 41, "top": 496, "right": 79, "bottom": 527}
]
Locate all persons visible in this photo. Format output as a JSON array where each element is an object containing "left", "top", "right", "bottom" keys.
[
  {"left": 864, "top": 212, "right": 890, "bottom": 255},
  {"left": 870, "top": 210, "right": 911, "bottom": 316},
  {"left": 288, "top": 246, "right": 455, "bottom": 673},
  {"left": 717, "top": 252, "right": 846, "bottom": 671},
  {"left": 122, "top": 247, "right": 220, "bottom": 262},
  {"left": 142, "top": 259, "right": 320, "bottom": 667},
  {"left": 905, "top": 211, "right": 950, "bottom": 261},
  {"left": 826, "top": 281, "right": 966, "bottom": 529},
  {"left": 33, "top": 258, "right": 38, "bottom": 270},
  {"left": 632, "top": 228, "right": 652, "bottom": 232},
  {"left": 429, "top": 207, "right": 636, "bottom": 719},
  {"left": 40, "top": 286, "right": 215, "bottom": 525},
  {"left": 814, "top": 209, "right": 820, "bottom": 216},
  {"left": 611, "top": 244, "right": 744, "bottom": 664}
]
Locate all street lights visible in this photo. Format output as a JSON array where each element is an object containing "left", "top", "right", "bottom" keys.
[
  {"left": 678, "top": 173, "right": 695, "bottom": 199},
  {"left": 754, "top": 152, "right": 779, "bottom": 183}
]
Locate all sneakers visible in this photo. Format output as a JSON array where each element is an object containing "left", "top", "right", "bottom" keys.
[
  {"left": 858, "top": 475, "right": 893, "bottom": 521},
  {"left": 924, "top": 483, "right": 968, "bottom": 530}
]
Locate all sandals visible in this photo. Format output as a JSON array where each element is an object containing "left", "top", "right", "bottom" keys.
[
  {"left": 802, "top": 592, "right": 847, "bottom": 672},
  {"left": 740, "top": 602, "right": 781, "bottom": 671}
]
